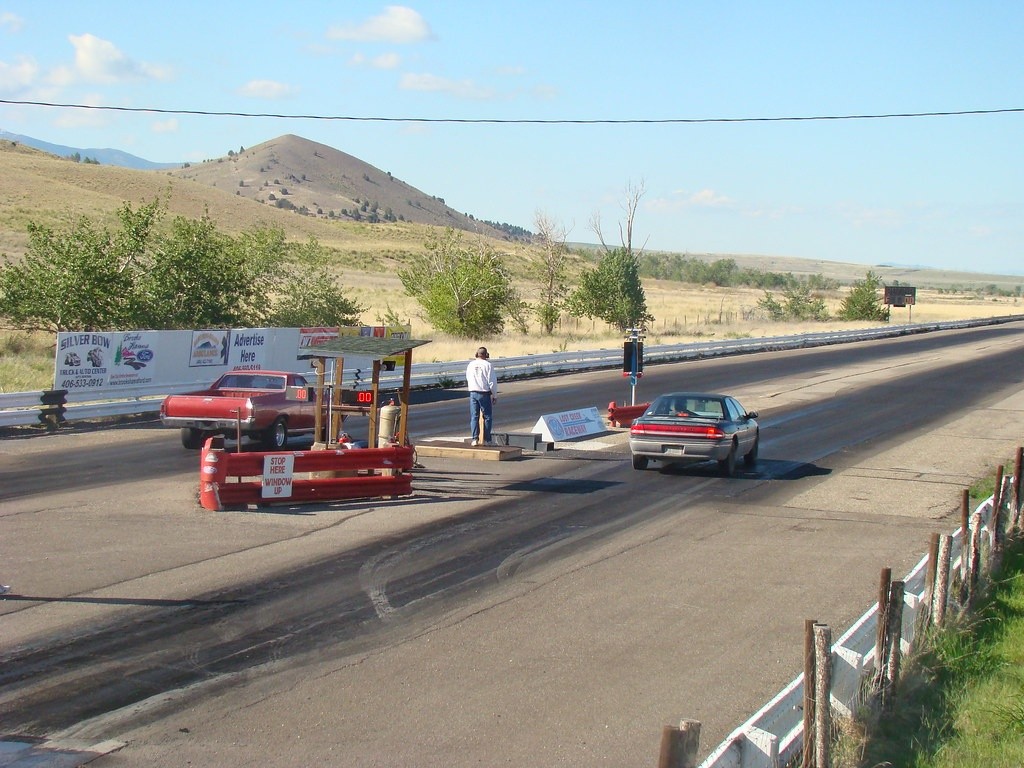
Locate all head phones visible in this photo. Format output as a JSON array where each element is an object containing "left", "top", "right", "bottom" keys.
[{"left": 475, "top": 352, "right": 489, "bottom": 358}]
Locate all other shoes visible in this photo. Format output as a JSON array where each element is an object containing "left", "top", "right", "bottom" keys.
[
  {"left": 484, "top": 441, "right": 498, "bottom": 446},
  {"left": 471, "top": 440, "right": 479, "bottom": 447}
]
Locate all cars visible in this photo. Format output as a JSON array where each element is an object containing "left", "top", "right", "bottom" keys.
[
  {"left": 628, "top": 393, "right": 763, "bottom": 475},
  {"left": 158, "top": 371, "right": 348, "bottom": 451}
]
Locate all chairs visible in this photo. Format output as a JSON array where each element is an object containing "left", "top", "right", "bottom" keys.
[
  {"left": 227, "top": 381, "right": 252, "bottom": 388},
  {"left": 265, "top": 383, "right": 282, "bottom": 389}
]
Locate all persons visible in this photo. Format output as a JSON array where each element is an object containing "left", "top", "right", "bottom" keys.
[
  {"left": 674, "top": 398, "right": 688, "bottom": 411},
  {"left": 466, "top": 347, "right": 497, "bottom": 446}
]
